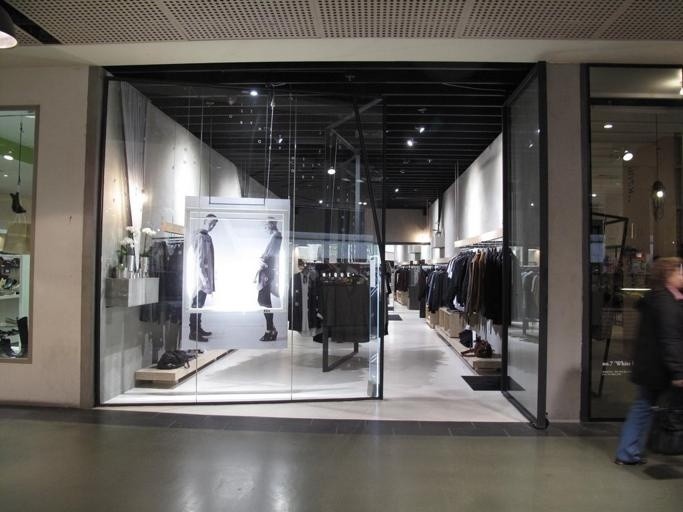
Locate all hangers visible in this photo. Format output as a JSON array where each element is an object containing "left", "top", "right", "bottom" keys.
[
  {"left": 521, "top": 264, "right": 541, "bottom": 273},
  {"left": 458, "top": 243, "right": 502, "bottom": 251},
  {"left": 298, "top": 263, "right": 354, "bottom": 275},
  {"left": 152, "top": 239, "right": 186, "bottom": 250}
]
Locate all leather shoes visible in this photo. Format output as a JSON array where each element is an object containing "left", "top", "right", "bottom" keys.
[{"left": 614, "top": 455, "right": 648, "bottom": 467}]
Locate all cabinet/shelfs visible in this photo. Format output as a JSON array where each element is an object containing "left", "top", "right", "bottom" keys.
[{"left": 0, "top": 254, "right": 30, "bottom": 354}]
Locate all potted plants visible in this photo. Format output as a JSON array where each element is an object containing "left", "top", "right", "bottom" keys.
[{"left": 115, "top": 264, "right": 127, "bottom": 278}]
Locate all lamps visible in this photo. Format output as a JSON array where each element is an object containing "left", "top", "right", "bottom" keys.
[
  {"left": 655, "top": 183, "right": 664, "bottom": 197},
  {"left": 0, "top": 4, "right": 19, "bottom": 49},
  {"left": 622, "top": 150, "right": 633, "bottom": 161}
]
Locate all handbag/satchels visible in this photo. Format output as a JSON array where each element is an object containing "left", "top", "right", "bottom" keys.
[
  {"left": 4, "top": 223, "right": 32, "bottom": 254},
  {"left": 650, "top": 408, "right": 683, "bottom": 457},
  {"left": 157, "top": 349, "right": 188, "bottom": 370},
  {"left": 474, "top": 340, "right": 493, "bottom": 358}
]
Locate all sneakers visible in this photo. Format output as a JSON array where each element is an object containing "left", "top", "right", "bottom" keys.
[{"left": 190, "top": 328, "right": 211, "bottom": 342}]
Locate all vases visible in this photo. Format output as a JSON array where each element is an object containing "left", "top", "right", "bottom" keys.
[{"left": 120, "top": 253, "right": 148, "bottom": 272}]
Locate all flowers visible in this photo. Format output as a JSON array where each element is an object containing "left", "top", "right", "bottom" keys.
[{"left": 120, "top": 226, "right": 155, "bottom": 252}]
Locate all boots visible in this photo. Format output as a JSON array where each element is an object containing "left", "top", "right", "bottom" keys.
[
  {"left": 11, "top": 193, "right": 26, "bottom": 212},
  {"left": 16, "top": 316, "right": 27, "bottom": 357},
  {"left": 0, "top": 338, "right": 17, "bottom": 357}
]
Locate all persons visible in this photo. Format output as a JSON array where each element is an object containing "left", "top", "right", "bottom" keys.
[
  {"left": 614, "top": 255, "right": 682, "bottom": 468},
  {"left": 186, "top": 212, "right": 218, "bottom": 342},
  {"left": 252, "top": 215, "right": 283, "bottom": 340}
]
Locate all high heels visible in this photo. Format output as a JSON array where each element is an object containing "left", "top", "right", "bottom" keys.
[{"left": 259, "top": 329, "right": 278, "bottom": 341}]
